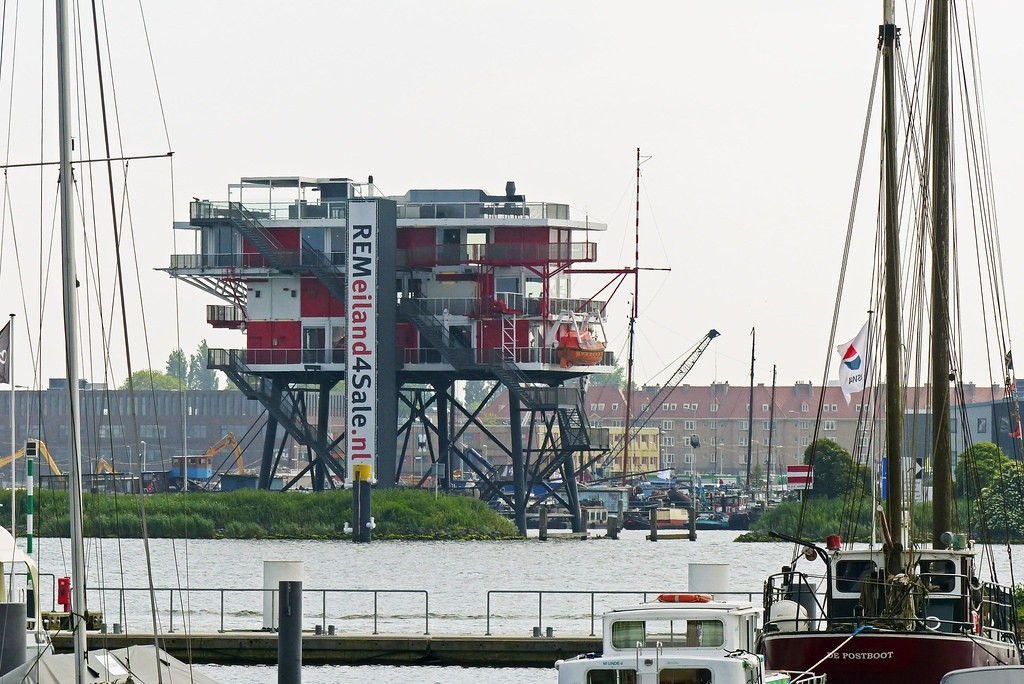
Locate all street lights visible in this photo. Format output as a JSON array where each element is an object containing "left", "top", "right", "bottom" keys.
[
  {"left": 140, "top": 441, "right": 146, "bottom": 471},
  {"left": 125, "top": 446, "right": 131, "bottom": 471}
]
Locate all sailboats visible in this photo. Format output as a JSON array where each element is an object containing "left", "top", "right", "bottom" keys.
[
  {"left": 0, "top": 0, "right": 220, "bottom": 684},
  {"left": 700, "top": 321, "right": 794, "bottom": 524},
  {"left": 755, "top": 1, "right": 1024, "bottom": 683}
]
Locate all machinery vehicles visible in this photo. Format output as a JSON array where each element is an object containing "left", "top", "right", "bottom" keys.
[
  {"left": 94, "top": 458, "right": 118, "bottom": 473},
  {"left": 0, "top": 438, "right": 68, "bottom": 488},
  {"left": 203, "top": 430, "right": 247, "bottom": 475}
]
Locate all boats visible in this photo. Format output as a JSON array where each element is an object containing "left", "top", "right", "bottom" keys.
[
  {"left": 488, "top": 492, "right": 580, "bottom": 528},
  {"left": 684, "top": 512, "right": 729, "bottom": 530},
  {"left": 554, "top": 565, "right": 829, "bottom": 684},
  {"left": 558, "top": 332, "right": 605, "bottom": 366},
  {"left": 622, "top": 507, "right": 659, "bottom": 530}
]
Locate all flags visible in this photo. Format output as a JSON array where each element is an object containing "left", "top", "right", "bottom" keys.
[
  {"left": 0, "top": 320, "right": 9, "bottom": 384},
  {"left": 836, "top": 321, "right": 878, "bottom": 406}
]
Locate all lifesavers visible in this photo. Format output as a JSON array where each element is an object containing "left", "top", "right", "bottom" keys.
[{"left": 658, "top": 593, "right": 712, "bottom": 603}]
